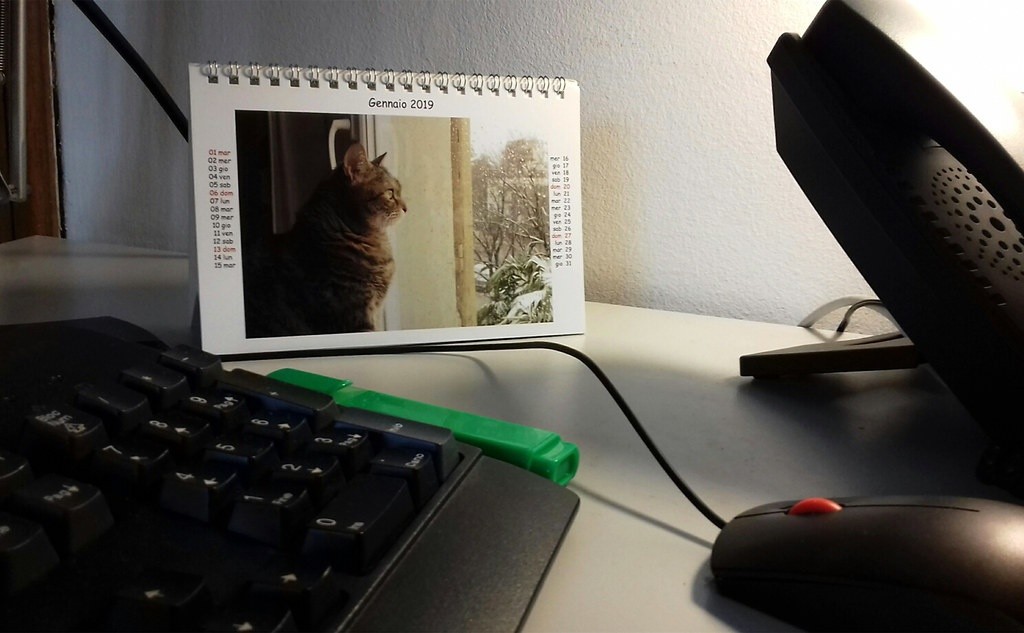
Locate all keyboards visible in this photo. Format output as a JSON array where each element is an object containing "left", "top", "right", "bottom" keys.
[{"left": 0, "top": 316, "right": 581, "bottom": 633}]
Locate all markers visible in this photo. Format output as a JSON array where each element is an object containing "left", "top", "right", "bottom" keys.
[{"left": 261, "top": 364, "right": 582, "bottom": 487}]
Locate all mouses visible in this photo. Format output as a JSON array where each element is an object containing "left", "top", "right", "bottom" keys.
[{"left": 710, "top": 491, "right": 1024, "bottom": 633}]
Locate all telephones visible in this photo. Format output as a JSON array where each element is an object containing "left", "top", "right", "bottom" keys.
[{"left": 763, "top": 1, "right": 1024, "bottom": 475}]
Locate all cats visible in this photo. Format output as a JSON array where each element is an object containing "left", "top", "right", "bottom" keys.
[{"left": 242, "top": 142, "right": 409, "bottom": 338}]
